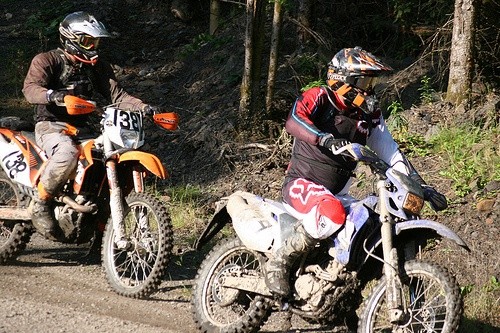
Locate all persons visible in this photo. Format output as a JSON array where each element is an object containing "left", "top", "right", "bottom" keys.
[
  {"left": 261, "top": 46, "right": 447, "bottom": 294},
  {"left": 21, "top": 11, "right": 161, "bottom": 239}
]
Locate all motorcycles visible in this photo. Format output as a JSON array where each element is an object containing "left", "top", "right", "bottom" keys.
[
  {"left": 190, "top": 138, "right": 471, "bottom": 333},
  {"left": 0, "top": 96, "right": 175, "bottom": 301}
]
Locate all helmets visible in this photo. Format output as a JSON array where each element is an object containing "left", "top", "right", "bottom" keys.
[
  {"left": 327, "top": 45, "right": 395, "bottom": 114},
  {"left": 58, "top": 11, "right": 110, "bottom": 59}
]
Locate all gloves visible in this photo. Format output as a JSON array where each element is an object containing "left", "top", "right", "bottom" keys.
[
  {"left": 143, "top": 103, "right": 168, "bottom": 115},
  {"left": 324, "top": 136, "right": 351, "bottom": 156},
  {"left": 49, "top": 91, "right": 86, "bottom": 103},
  {"left": 423, "top": 189, "right": 448, "bottom": 211}
]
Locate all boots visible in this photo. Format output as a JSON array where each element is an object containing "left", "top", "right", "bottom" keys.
[{"left": 264, "top": 222, "right": 320, "bottom": 295}]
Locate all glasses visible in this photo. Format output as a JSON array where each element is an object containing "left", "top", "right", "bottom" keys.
[
  {"left": 350, "top": 74, "right": 381, "bottom": 95},
  {"left": 77, "top": 34, "right": 101, "bottom": 50}
]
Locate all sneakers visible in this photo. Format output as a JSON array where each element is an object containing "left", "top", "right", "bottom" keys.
[{"left": 28, "top": 196, "right": 57, "bottom": 232}]
